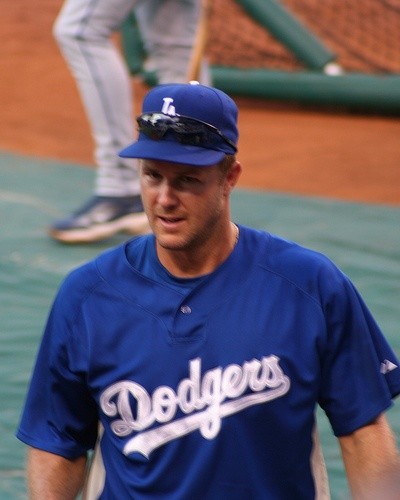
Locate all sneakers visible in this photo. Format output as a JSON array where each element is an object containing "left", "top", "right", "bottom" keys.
[{"left": 47, "top": 196, "right": 150, "bottom": 243}]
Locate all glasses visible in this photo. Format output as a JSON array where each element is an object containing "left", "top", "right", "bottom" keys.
[{"left": 136, "top": 112, "right": 238, "bottom": 152}]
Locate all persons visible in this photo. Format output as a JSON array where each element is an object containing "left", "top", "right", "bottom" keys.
[
  {"left": 47, "top": 0, "right": 214, "bottom": 245},
  {"left": 15, "top": 81, "right": 400, "bottom": 500}
]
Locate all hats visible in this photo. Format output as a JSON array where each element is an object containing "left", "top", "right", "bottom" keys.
[{"left": 119, "top": 81, "right": 239, "bottom": 166}]
